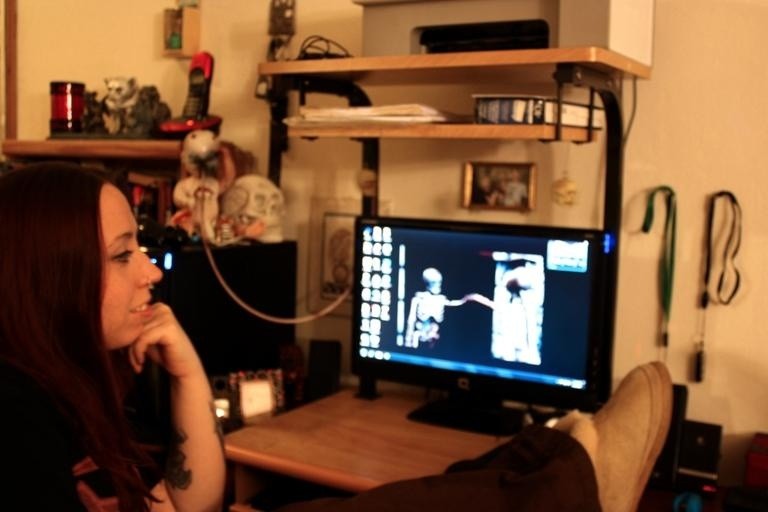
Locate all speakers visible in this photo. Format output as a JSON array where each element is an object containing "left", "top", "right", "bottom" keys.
[
  {"left": 301, "top": 339, "right": 343, "bottom": 404},
  {"left": 648, "top": 385, "right": 687, "bottom": 490}
]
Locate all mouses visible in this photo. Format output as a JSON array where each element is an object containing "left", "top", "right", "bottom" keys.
[{"left": 672, "top": 491, "right": 704, "bottom": 512}]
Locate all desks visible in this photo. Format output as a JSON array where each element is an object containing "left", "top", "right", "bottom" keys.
[{"left": 218, "top": 373, "right": 569, "bottom": 503}]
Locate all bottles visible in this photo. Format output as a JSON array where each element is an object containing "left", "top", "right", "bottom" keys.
[
  {"left": 130, "top": 184, "right": 145, "bottom": 222},
  {"left": 169, "top": 18, "right": 182, "bottom": 50}
]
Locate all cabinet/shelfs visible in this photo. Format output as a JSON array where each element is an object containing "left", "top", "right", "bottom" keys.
[
  {"left": 0, "top": 136, "right": 219, "bottom": 436},
  {"left": 258, "top": 46, "right": 650, "bottom": 142}
]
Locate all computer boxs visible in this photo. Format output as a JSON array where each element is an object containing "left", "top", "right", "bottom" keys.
[{"left": 147, "top": 238, "right": 298, "bottom": 377}]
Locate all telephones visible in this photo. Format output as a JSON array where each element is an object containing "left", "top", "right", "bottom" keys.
[{"left": 158, "top": 51, "right": 223, "bottom": 139}]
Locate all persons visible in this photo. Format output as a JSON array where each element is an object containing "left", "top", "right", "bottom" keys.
[
  {"left": 471, "top": 168, "right": 528, "bottom": 207},
  {"left": 258, "top": 361, "right": 672, "bottom": 512},
  {"left": 0, "top": 165, "right": 227, "bottom": 512}
]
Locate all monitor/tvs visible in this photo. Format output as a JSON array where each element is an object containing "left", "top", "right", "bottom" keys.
[{"left": 348, "top": 214, "right": 619, "bottom": 437}]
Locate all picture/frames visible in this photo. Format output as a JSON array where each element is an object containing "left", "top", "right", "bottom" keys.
[
  {"left": 462, "top": 160, "right": 536, "bottom": 211},
  {"left": 305, "top": 195, "right": 391, "bottom": 317}
]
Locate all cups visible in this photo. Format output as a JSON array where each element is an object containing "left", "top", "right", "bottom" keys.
[{"left": 49, "top": 81, "right": 88, "bottom": 134}]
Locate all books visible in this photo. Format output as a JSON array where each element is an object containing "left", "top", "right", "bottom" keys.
[{"left": 282, "top": 103, "right": 465, "bottom": 129}]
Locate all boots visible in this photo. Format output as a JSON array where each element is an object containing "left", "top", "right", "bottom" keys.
[{"left": 552, "top": 362, "right": 673, "bottom": 512}]
